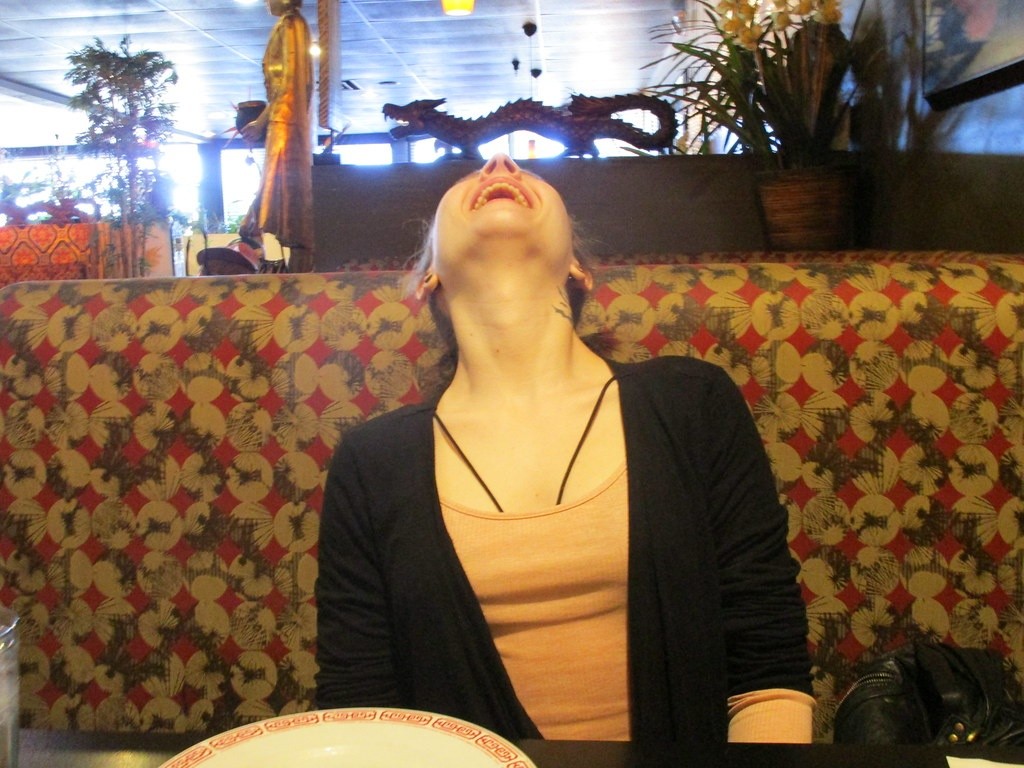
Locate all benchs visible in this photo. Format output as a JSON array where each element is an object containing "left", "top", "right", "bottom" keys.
[
  {"left": 0, "top": 249, "right": 1024, "bottom": 732},
  {"left": 0, "top": 222, "right": 134, "bottom": 292}
]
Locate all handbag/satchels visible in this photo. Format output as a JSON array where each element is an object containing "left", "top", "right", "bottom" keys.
[{"left": 834, "top": 642, "right": 1024, "bottom": 750}]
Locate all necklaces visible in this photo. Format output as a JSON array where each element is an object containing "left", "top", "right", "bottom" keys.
[{"left": 433, "top": 374, "right": 617, "bottom": 512}]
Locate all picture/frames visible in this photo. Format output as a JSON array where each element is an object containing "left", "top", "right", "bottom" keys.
[{"left": 921, "top": 0, "right": 1024, "bottom": 112}]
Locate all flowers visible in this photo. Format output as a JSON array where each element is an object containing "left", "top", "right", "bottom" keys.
[{"left": 636, "top": 0, "right": 863, "bottom": 164}]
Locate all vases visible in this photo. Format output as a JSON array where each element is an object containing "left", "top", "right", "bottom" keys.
[{"left": 754, "top": 169, "right": 840, "bottom": 249}]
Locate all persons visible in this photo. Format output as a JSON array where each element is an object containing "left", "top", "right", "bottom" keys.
[
  {"left": 317, "top": 153, "right": 817, "bottom": 745},
  {"left": 242, "top": 0, "right": 315, "bottom": 274}
]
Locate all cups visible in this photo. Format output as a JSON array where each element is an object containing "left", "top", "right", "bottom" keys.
[{"left": 0, "top": 606, "right": 22, "bottom": 768}]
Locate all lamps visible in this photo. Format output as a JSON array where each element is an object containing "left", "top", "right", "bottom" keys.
[{"left": 442, "top": 0, "right": 475, "bottom": 17}]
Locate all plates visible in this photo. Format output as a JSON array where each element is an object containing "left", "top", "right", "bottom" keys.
[{"left": 158, "top": 707, "right": 538, "bottom": 768}]
